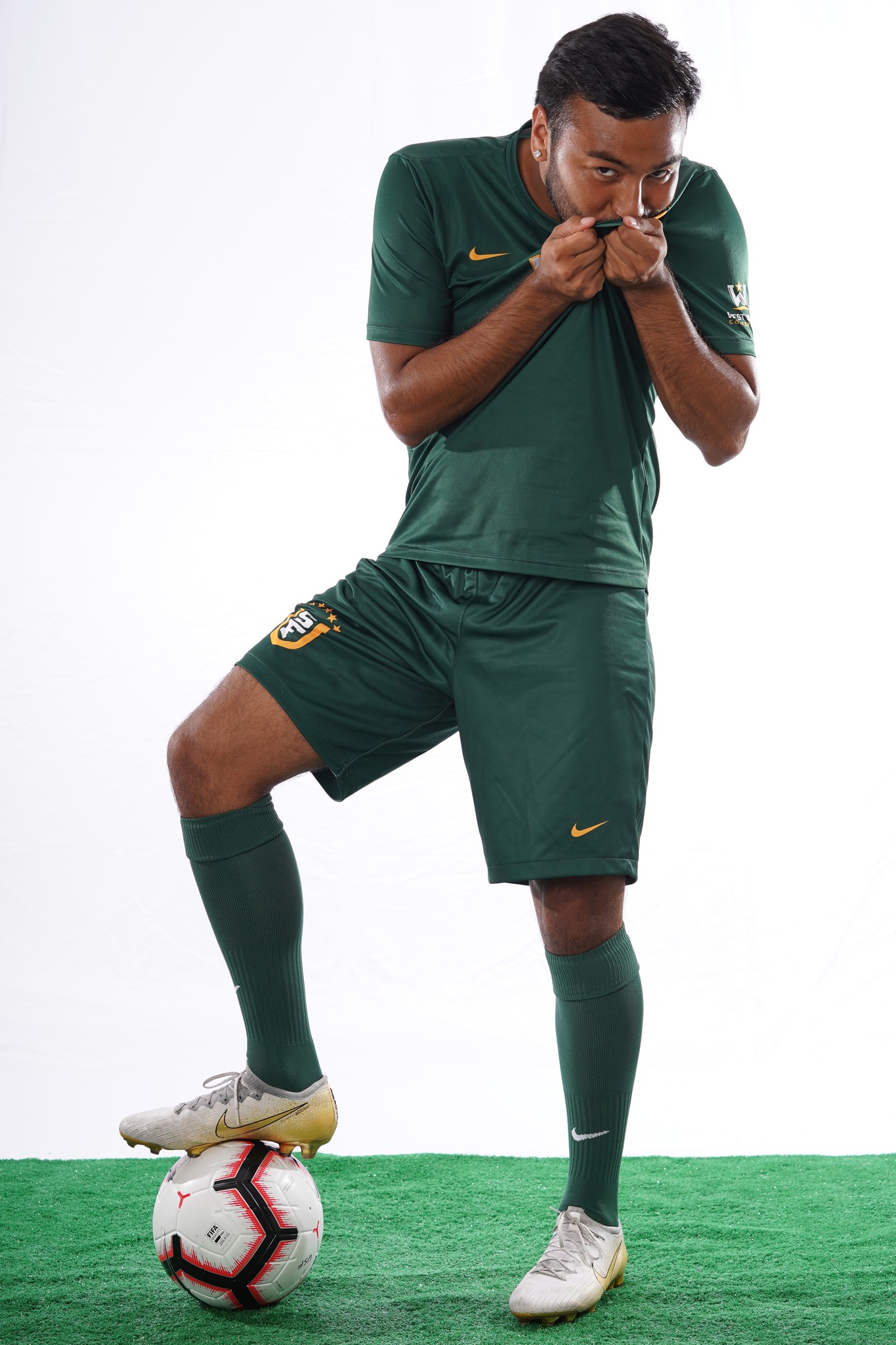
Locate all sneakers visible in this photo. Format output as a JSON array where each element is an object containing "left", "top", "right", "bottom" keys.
[
  {"left": 118, "top": 1060, "right": 338, "bottom": 1158},
  {"left": 509, "top": 1205, "right": 627, "bottom": 1325}
]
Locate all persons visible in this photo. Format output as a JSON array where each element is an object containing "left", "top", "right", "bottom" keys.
[{"left": 121, "top": 8, "right": 758, "bottom": 1321}]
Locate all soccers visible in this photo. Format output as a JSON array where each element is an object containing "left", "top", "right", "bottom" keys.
[{"left": 151, "top": 1137, "right": 324, "bottom": 1313}]
